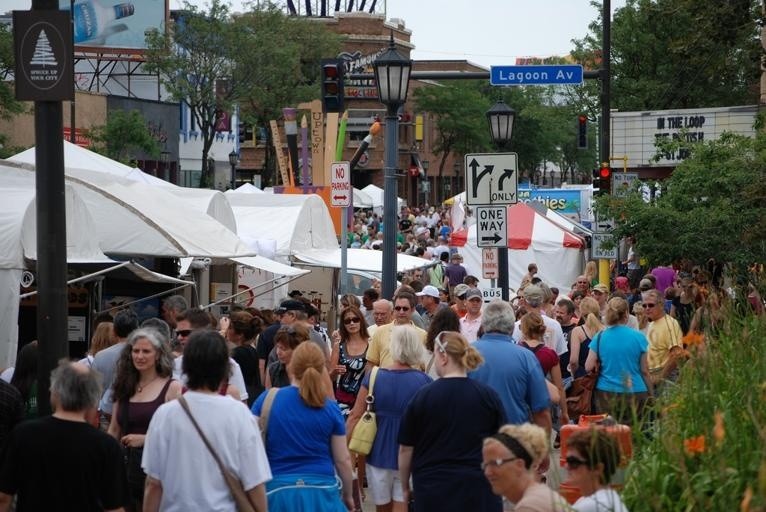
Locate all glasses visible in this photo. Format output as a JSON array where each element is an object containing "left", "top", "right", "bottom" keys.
[
  {"left": 344, "top": 317, "right": 361, "bottom": 325},
  {"left": 478, "top": 456, "right": 524, "bottom": 469},
  {"left": 639, "top": 301, "right": 662, "bottom": 308},
  {"left": 563, "top": 454, "right": 589, "bottom": 470},
  {"left": 393, "top": 306, "right": 411, "bottom": 313},
  {"left": 175, "top": 328, "right": 192, "bottom": 338}
]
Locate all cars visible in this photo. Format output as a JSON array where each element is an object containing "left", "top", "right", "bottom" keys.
[{"left": 336, "top": 268, "right": 401, "bottom": 315}]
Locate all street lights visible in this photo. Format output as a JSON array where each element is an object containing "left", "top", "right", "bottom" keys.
[
  {"left": 160, "top": 145, "right": 170, "bottom": 180},
  {"left": 229, "top": 148, "right": 238, "bottom": 189},
  {"left": 455, "top": 161, "right": 460, "bottom": 193},
  {"left": 373, "top": 30, "right": 414, "bottom": 302},
  {"left": 550, "top": 168, "right": 555, "bottom": 188},
  {"left": 535, "top": 168, "right": 541, "bottom": 184},
  {"left": 423, "top": 157, "right": 430, "bottom": 210},
  {"left": 487, "top": 88, "right": 517, "bottom": 301}
]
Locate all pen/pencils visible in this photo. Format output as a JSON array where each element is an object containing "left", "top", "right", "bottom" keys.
[
  {"left": 301, "top": 115, "right": 309, "bottom": 187},
  {"left": 338, "top": 110, "right": 349, "bottom": 164}
]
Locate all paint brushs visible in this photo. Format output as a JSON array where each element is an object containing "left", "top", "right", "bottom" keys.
[
  {"left": 284, "top": 108, "right": 300, "bottom": 187},
  {"left": 345, "top": 122, "right": 382, "bottom": 173}
]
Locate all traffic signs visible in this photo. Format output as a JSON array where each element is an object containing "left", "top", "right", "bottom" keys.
[
  {"left": 477, "top": 207, "right": 506, "bottom": 247},
  {"left": 491, "top": 65, "right": 582, "bottom": 86},
  {"left": 464, "top": 152, "right": 518, "bottom": 204},
  {"left": 597, "top": 209, "right": 614, "bottom": 231},
  {"left": 591, "top": 234, "right": 618, "bottom": 258}
]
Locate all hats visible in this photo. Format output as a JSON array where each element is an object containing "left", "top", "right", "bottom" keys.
[
  {"left": 591, "top": 284, "right": 609, "bottom": 294},
  {"left": 638, "top": 277, "right": 653, "bottom": 291},
  {"left": 454, "top": 283, "right": 483, "bottom": 302},
  {"left": 272, "top": 299, "right": 305, "bottom": 315},
  {"left": 415, "top": 284, "right": 442, "bottom": 300}
]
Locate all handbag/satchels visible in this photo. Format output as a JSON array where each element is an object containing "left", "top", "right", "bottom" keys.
[
  {"left": 219, "top": 467, "right": 258, "bottom": 512},
  {"left": 563, "top": 371, "right": 598, "bottom": 420},
  {"left": 349, "top": 407, "right": 377, "bottom": 455},
  {"left": 122, "top": 446, "right": 150, "bottom": 485}
]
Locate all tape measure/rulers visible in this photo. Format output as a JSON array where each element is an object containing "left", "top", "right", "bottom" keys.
[{"left": 271, "top": 120, "right": 288, "bottom": 185}]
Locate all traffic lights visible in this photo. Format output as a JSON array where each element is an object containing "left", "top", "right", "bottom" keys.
[
  {"left": 593, "top": 167, "right": 600, "bottom": 198},
  {"left": 600, "top": 168, "right": 611, "bottom": 197},
  {"left": 322, "top": 59, "right": 344, "bottom": 112},
  {"left": 579, "top": 115, "right": 587, "bottom": 147}
]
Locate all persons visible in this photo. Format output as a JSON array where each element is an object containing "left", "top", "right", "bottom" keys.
[{"left": 1, "top": 184, "right": 766, "bottom": 511}]
